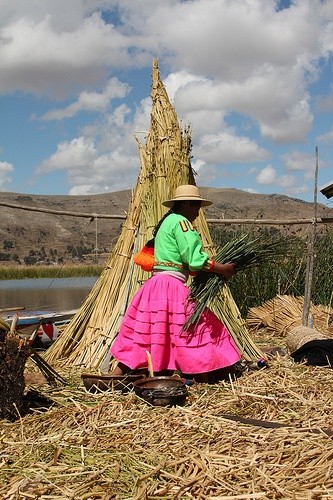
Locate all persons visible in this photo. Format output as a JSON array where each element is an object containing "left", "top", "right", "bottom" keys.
[{"left": 110, "top": 185, "right": 241, "bottom": 379}]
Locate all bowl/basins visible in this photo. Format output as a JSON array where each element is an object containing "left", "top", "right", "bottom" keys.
[
  {"left": 133, "top": 377, "right": 188, "bottom": 408},
  {"left": 81, "top": 373, "right": 143, "bottom": 396}
]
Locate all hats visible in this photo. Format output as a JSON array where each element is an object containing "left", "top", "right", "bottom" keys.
[{"left": 161, "top": 184, "right": 211, "bottom": 209}]
